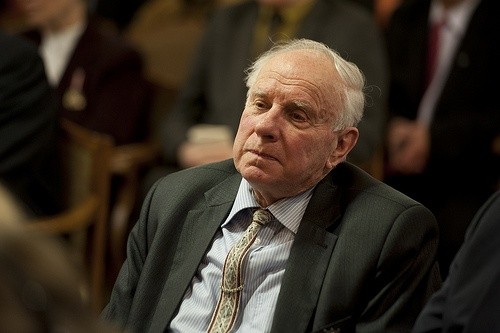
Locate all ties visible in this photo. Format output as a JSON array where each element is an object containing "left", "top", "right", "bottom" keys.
[
  {"left": 204, "top": 209, "right": 273, "bottom": 333},
  {"left": 421, "top": 20, "right": 447, "bottom": 99}
]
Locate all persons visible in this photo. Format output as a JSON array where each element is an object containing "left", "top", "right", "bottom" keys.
[
  {"left": 1, "top": 1, "right": 500, "bottom": 333},
  {"left": 98, "top": 38, "right": 442, "bottom": 333}
]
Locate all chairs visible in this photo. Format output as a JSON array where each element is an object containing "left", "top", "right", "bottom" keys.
[{"left": 31, "top": 119, "right": 112, "bottom": 318}]
